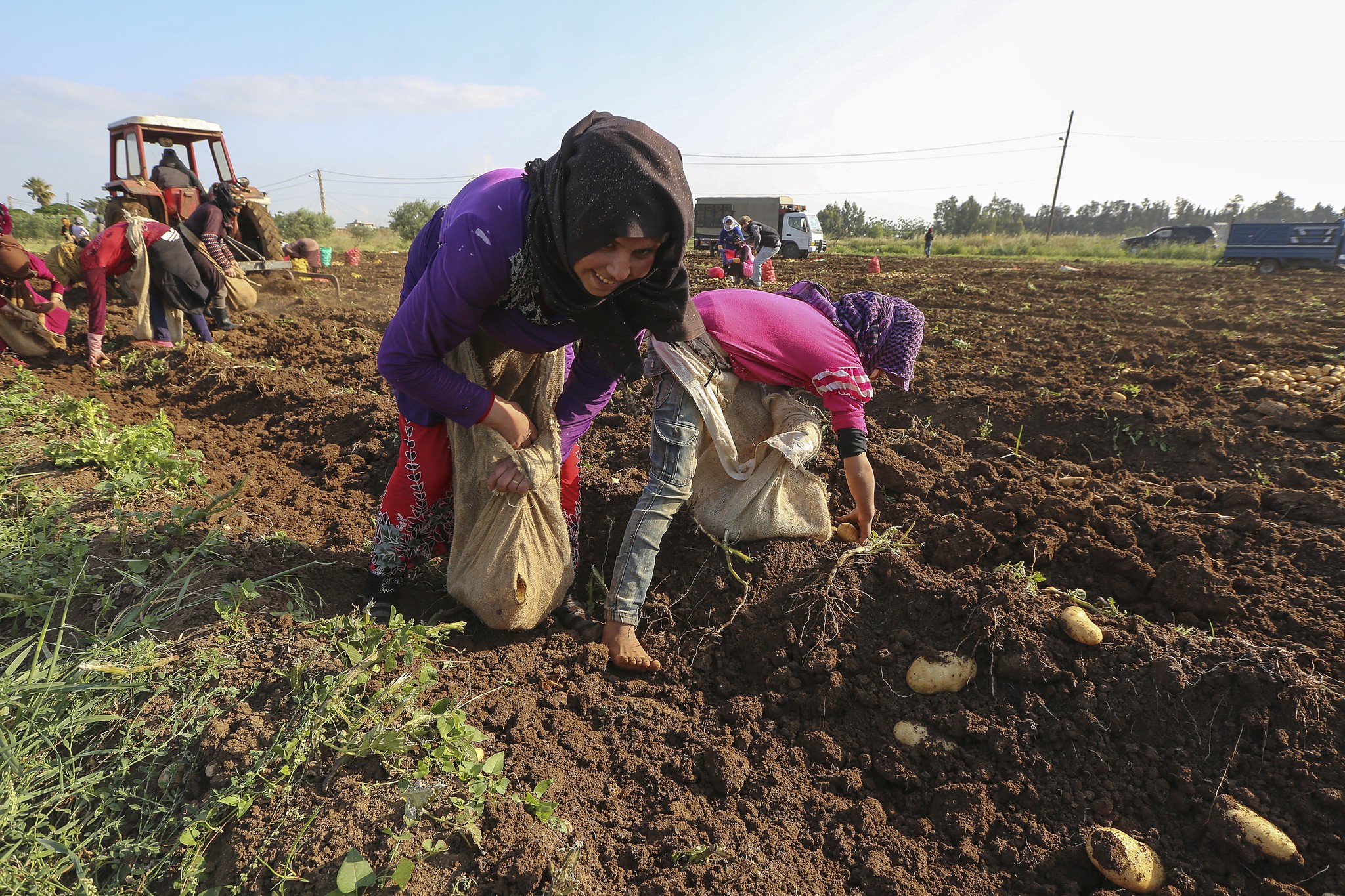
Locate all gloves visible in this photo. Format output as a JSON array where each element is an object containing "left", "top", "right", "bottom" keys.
[
  {"left": 719, "top": 249, "right": 728, "bottom": 254},
  {"left": 724, "top": 259, "right": 733, "bottom": 265},
  {"left": 86, "top": 332, "right": 111, "bottom": 373}
]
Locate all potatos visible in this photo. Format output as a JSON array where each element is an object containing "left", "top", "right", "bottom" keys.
[
  {"left": 1060, "top": 605, "right": 1103, "bottom": 645},
  {"left": 1087, "top": 827, "right": 1163, "bottom": 895},
  {"left": 893, "top": 721, "right": 958, "bottom": 756},
  {"left": 1059, "top": 477, "right": 1086, "bottom": 488},
  {"left": 100, "top": 360, "right": 111, "bottom": 368},
  {"left": 1238, "top": 364, "right": 1345, "bottom": 396},
  {"left": 837, "top": 523, "right": 858, "bottom": 543},
  {"left": 1208, "top": 795, "right": 1297, "bottom": 864},
  {"left": 1110, "top": 391, "right": 1126, "bottom": 401},
  {"left": 906, "top": 652, "right": 977, "bottom": 693}
]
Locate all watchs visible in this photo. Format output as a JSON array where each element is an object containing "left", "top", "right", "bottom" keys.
[{"left": 720, "top": 248, "right": 723, "bottom": 250}]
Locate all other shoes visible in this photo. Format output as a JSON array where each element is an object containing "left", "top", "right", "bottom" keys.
[{"left": 722, "top": 268, "right": 760, "bottom": 287}]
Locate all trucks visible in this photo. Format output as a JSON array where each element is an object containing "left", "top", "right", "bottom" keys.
[
  {"left": 1218, "top": 221, "right": 1345, "bottom": 277},
  {"left": 693, "top": 196, "right": 827, "bottom": 258}
]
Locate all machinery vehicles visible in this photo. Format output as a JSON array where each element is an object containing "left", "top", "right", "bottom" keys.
[{"left": 102, "top": 116, "right": 294, "bottom": 274}]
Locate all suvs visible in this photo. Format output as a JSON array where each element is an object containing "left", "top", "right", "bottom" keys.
[{"left": 1118, "top": 225, "right": 1217, "bottom": 253}]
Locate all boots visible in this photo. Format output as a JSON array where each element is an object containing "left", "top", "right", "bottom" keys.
[{"left": 211, "top": 307, "right": 244, "bottom": 329}]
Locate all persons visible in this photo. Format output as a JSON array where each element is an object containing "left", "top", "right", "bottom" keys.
[
  {"left": 0, "top": 202, "right": 14, "bottom": 236},
  {"left": 177, "top": 180, "right": 246, "bottom": 332},
  {"left": 0, "top": 233, "right": 68, "bottom": 368},
  {"left": 94, "top": 215, "right": 107, "bottom": 234},
  {"left": 282, "top": 237, "right": 320, "bottom": 275},
  {"left": 43, "top": 215, "right": 217, "bottom": 370},
  {"left": 360, "top": 110, "right": 700, "bottom": 628},
  {"left": 150, "top": 148, "right": 198, "bottom": 191},
  {"left": 67, "top": 215, "right": 91, "bottom": 247},
  {"left": 60, "top": 217, "right": 75, "bottom": 242},
  {"left": 924, "top": 228, "right": 935, "bottom": 258},
  {"left": 739, "top": 215, "right": 782, "bottom": 287},
  {"left": 600, "top": 279, "right": 928, "bottom": 674},
  {"left": 724, "top": 234, "right": 755, "bottom": 285},
  {"left": 716, "top": 215, "right": 747, "bottom": 276}
]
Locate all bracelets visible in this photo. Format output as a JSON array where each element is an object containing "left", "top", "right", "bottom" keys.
[{"left": 742, "top": 240, "right": 746, "bottom": 244}]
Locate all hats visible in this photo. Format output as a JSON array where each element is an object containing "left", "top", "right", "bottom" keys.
[{"left": 738, "top": 215, "right": 751, "bottom": 224}]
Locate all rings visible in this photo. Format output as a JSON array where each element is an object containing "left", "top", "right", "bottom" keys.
[{"left": 511, "top": 479, "right": 519, "bottom": 486}]
